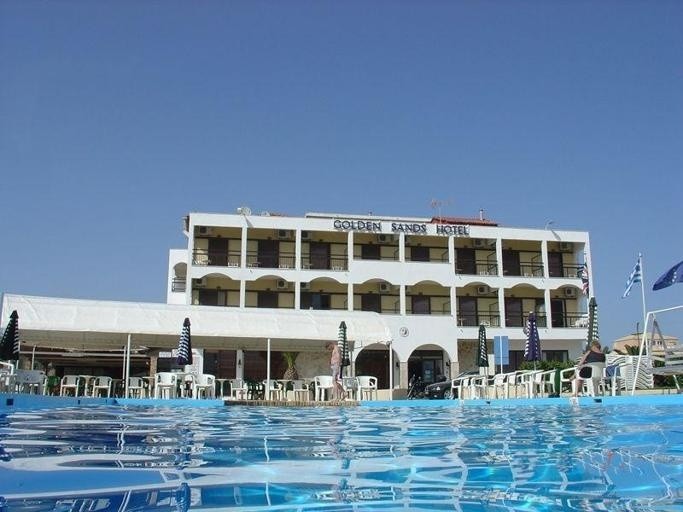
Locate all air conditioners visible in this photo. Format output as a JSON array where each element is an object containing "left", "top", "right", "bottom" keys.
[
  {"left": 473, "top": 238, "right": 486, "bottom": 248},
  {"left": 194, "top": 277, "right": 206, "bottom": 287},
  {"left": 559, "top": 242, "right": 574, "bottom": 253},
  {"left": 301, "top": 230, "right": 312, "bottom": 242},
  {"left": 300, "top": 282, "right": 311, "bottom": 291},
  {"left": 377, "top": 234, "right": 393, "bottom": 244},
  {"left": 405, "top": 235, "right": 409, "bottom": 242},
  {"left": 199, "top": 226, "right": 213, "bottom": 236},
  {"left": 564, "top": 285, "right": 577, "bottom": 299},
  {"left": 379, "top": 283, "right": 390, "bottom": 291},
  {"left": 277, "top": 229, "right": 293, "bottom": 241},
  {"left": 405, "top": 285, "right": 412, "bottom": 292},
  {"left": 477, "top": 285, "right": 490, "bottom": 295},
  {"left": 276, "top": 279, "right": 289, "bottom": 289}
]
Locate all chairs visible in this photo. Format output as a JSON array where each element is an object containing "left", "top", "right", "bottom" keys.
[
  {"left": 1, "top": 362, "right": 379, "bottom": 403},
  {"left": 451, "top": 356, "right": 635, "bottom": 399}
]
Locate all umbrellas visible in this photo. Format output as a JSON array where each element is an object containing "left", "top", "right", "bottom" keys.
[
  {"left": 524, "top": 311, "right": 542, "bottom": 371},
  {"left": 585, "top": 300, "right": 600, "bottom": 353},
  {"left": 0, "top": 310, "right": 22, "bottom": 373},
  {"left": 476, "top": 324, "right": 490, "bottom": 376},
  {"left": 336, "top": 321, "right": 350, "bottom": 380},
  {"left": 177, "top": 315, "right": 193, "bottom": 398}
]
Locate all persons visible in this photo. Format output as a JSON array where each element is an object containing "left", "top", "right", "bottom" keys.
[
  {"left": 570, "top": 339, "right": 604, "bottom": 397},
  {"left": 325, "top": 342, "right": 346, "bottom": 401}
]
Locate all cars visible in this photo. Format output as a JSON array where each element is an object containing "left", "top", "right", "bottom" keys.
[{"left": 424, "top": 366, "right": 496, "bottom": 399}]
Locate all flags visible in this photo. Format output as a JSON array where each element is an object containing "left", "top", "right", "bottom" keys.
[
  {"left": 582, "top": 254, "right": 589, "bottom": 299},
  {"left": 620, "top": 260, "right": 641, "bottom": 300},
  {"left": 651, "top": 258, "right": 683, "bottom": 291}
]
